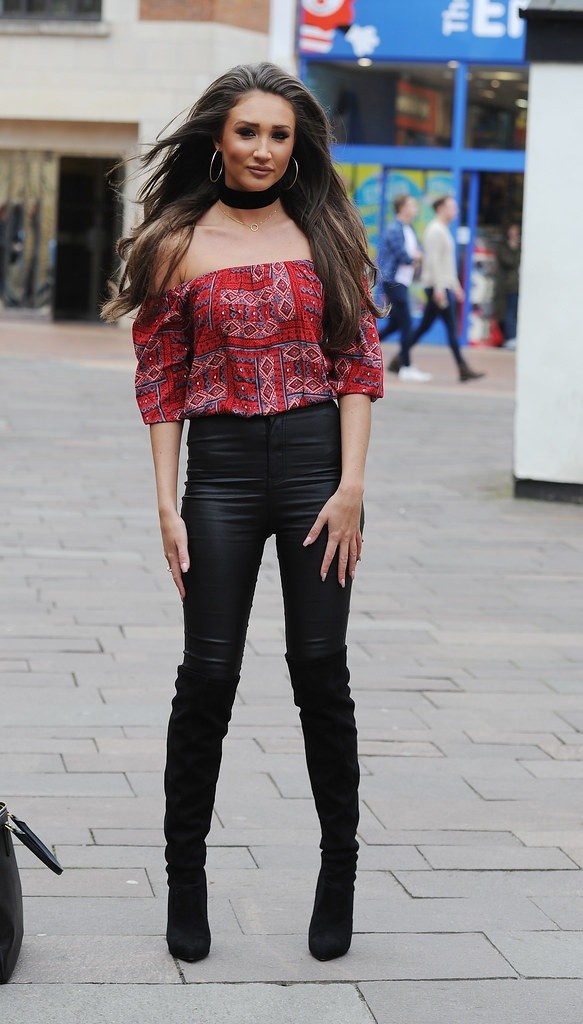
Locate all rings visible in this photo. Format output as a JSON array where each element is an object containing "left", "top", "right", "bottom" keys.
[{"left": 166, "top": 567, "right": 172, "bottom": 572}]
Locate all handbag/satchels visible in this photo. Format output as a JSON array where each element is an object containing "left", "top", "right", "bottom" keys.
[{"left": 0, "top": 802, "right": 64, "bottom": 984}]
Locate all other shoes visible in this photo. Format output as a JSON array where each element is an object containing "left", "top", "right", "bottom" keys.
[
  {"left": 460, "top": 369, "right": 486, "bottom": 383},
  {"left": 390, "top": 357, "right": 404, "bottom": 371},
  {"left": 397, "top": 368, "right": 430, "bottom": 383}
]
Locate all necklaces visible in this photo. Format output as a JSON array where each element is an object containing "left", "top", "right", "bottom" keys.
[{"left": 216, "top": 201, "right": 282, "bottom": 232}]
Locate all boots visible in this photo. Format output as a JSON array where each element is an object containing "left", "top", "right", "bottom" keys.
[
  {"left": 285, "top": 645, "right": 361, "bottom": 962},
  {"left": 164, "top": 665, "right": 241, "bottom": 963}
]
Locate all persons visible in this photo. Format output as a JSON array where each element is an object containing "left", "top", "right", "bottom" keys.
[
  {"left": 100, "top": 64, "right": 392, "bottom": 962},
  {"left": 495, "top": 222, "right": 520, "bottom": 350},
  {"left": 375, "top": 193, "right": 484, "bottom": 382}
]
